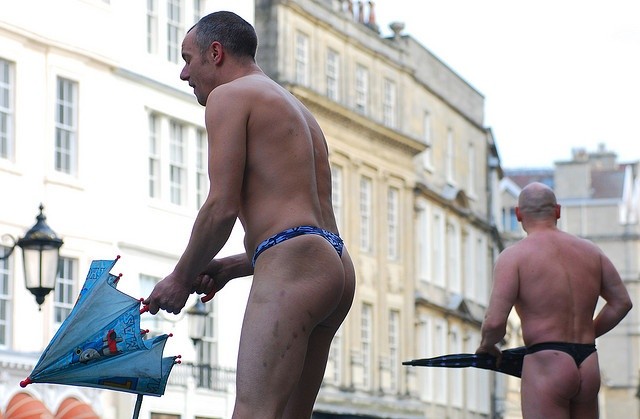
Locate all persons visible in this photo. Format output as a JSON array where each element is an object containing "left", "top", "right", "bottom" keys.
[
  {"left": 476, "top": 182, "right": 633, "bottom": 419},
  {"left": 143, "top": 12, "right": 356, "bottom": 419}
]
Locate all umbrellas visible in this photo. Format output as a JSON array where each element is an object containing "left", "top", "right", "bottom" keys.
[
  {"left": 19, "top": 256, "right": 221, "bottom": 397},
  {"left": 401, "top": 344, "right": 527, "bottom": 381}
]
